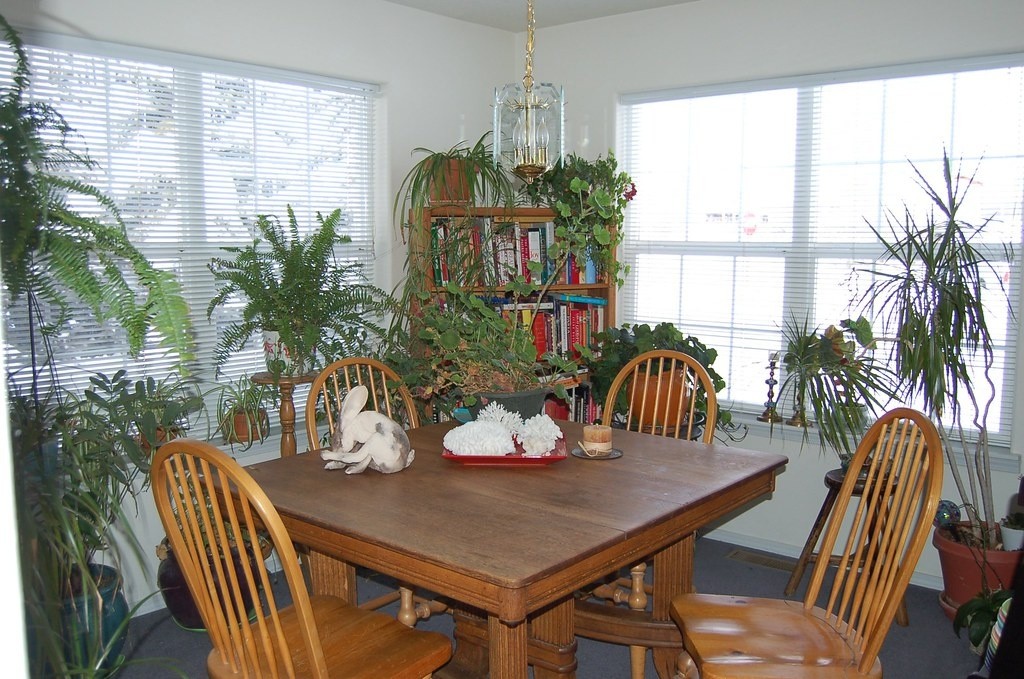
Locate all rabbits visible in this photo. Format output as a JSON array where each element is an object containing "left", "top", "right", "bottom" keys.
[{"left": 319, "top": 384, "right": 416, "bottom": 475}]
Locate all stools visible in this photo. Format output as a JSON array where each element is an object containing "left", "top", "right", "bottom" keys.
[{"left": 785, "top": 468, "right": 914, "bottom": 627}]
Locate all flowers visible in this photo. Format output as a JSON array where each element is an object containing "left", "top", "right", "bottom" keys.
[{"left": 528, "top": 150, "right": 637, "bottom": 291}]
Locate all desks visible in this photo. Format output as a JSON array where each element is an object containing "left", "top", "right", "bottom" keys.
[
  {"left": 250, "top": 372, "right": 319, "bottom": 458},
  {"left": 612, "top": 423, "right": 702, "bottom": 443}
]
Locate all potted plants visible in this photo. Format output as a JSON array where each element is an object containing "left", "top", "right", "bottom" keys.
[
  {"left": 204, "top": 203, "right": 405, "bottom": 378},
  {"left": 414, "top": 176, "right": 615, "bottom": 424},
  {"left": 769, "top": 146, "right": 1024, "bottom": 625},
  {"left": 392, "top": 130, "right": 529, "bottom": 245},
  {"left": 156, "top": 470, "right": 273, "bottom": 631},
  {"left": 115, "top": 372, "right": 210, "bottom": 456},
  {"left": 783, "top": 315, "right": 878, "bottom": 434},
  {"left": 7, "top": 368, "right": 203, "bottom": 679},
  {"left": 59, "top": 380, "right": 111, "bottom": 461},
  {"left": 198, "top": 374, "right": 273, "bottom": 454},
  {"left": 999, "top": 513, "right": 1024, "bottom": 551},
  {"left": 574, "top": 323, "right": 749, "bottom": 447}
]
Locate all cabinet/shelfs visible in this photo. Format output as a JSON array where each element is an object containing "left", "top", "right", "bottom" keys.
[{"left": 409, "top": 206, "right": 616, "bottom": 424}]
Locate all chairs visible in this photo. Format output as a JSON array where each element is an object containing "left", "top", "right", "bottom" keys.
[
  {"left": 305, "top": 358, "right": 453, "bottom": 629},
  {"left": 150, "top": 438, "right": 452, "bottom": 679},
  {"left": 669, "top": 407, "right": 943, "bottom": 679},
  {"left": 573, "top": 350, "right": 717, "bottom": 679}
]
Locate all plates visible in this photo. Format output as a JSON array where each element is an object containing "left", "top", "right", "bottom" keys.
[{"left": 442, "top": 431, "right": 570, "bottom": 468}]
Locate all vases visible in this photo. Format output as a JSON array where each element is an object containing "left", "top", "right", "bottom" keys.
[
  {"left": 560, "top": 180, "right": 589, "bottom": 206},
  {"left": 839, "top": 455, "right": 892, "bottom": 480}
]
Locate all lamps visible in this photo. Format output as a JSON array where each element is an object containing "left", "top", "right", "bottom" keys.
[{"left": 493, "top": 0, "right": 565, "bottom": 184}]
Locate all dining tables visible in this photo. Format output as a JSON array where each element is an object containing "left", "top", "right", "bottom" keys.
[{"left": 192, "top": 417, "right": 788, "bottom": 679}]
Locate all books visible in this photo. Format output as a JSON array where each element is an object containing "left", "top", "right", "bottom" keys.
[
  {"left": 429, "top": 384, "right": 465, "bottom": 427},
  {"left": 439, "top": 292, "right": 608, "bottom": 389},
  {"left": 429, "top": 218, "right": 611, "bottom": 284},
  {"left": 538, "top": 389, "right": 606, "bottom": 425}
]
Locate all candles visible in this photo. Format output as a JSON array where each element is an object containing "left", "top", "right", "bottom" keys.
[{"left": 583, "top": 423, "right": 613, "bottom": 456}]
